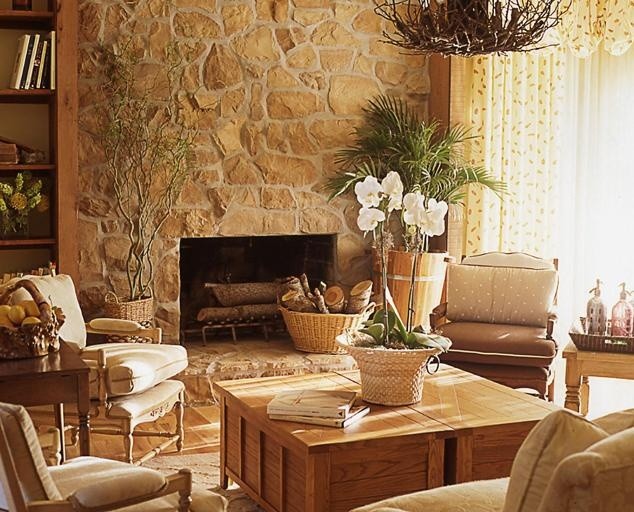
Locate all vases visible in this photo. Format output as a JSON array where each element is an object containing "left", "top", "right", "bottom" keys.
[{"left": 6, "top": 214, "right": 29, "bottom": 239}]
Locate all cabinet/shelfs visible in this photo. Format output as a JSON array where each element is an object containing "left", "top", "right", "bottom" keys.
[{"left": 0, "top": 0, "right": 81, "bottom": 301}]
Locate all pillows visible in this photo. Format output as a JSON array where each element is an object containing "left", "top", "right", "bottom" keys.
[
  {"left": 504, "top": 407, "right": 634, "bottom": 512},
  {"left": 78, "top": 343, "right": 189, "bottom": 402},
  {"left": 444, "top": 260, "right": 558, "bottom": 328}
]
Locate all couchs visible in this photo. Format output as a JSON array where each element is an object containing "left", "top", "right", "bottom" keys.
[
  {"left": 2, "top": 272, "right": 192, "bottom": 467},
  {"left": 347, "top": 426, "right": 634, "bottom": 512},
  {"left": 0, "top": 403, "right": 230, "bottom": 512},
  {"left": 428, "top": 250, "right": 560, "bottom": 404}
]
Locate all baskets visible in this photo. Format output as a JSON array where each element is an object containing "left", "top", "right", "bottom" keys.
[
  {"left": 0, "top": 279, "right": 66, "bottom": 359},
  {"left": 104, "top": 284, "right": 154, "bottom": 343},
  {"left": 276, "top": 294, "right": 376, "bottom": 354},
  {"left": 565, "top": 306, "right": 634, "bottom": 355}
]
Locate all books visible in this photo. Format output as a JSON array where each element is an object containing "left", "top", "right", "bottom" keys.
[
  {"left": 269, "top": 405, "right": 371, "bottom": 428},
  {"left": 10, "top": 30, "right": 55, "bottom": 91},
  {"left": 266, "top": 390, "right": 357, "bottom": 417}
]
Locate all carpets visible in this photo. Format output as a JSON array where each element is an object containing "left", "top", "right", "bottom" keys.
[{"left": 140, "top": 446, "right": 281, "bottom": 512}]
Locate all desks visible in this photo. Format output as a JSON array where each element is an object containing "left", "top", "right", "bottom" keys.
[
  {"left": 562, "top": 340, "right": 634, "bottom": 417},
  {"left": 1, "top": 336, "right": 92, "bottom": 466}
]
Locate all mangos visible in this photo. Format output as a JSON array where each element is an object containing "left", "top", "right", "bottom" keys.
[{"left": 0, "top": 299, "right": 39, "bottom": 331}]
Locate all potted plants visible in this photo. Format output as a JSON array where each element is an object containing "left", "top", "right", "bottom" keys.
[
  {"left": 82, "top": 30, "right": 221, "bottom": 342},
  {"left": 318, "top": 92, "right": 513, "bottom": 332}
]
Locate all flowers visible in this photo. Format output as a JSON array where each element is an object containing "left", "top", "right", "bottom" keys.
[
  {"left": 355, "top": 170, "right": 454, "bottom": 352},
  {"left": 0, "top": 171, "right": 51, "bottom": 236}
]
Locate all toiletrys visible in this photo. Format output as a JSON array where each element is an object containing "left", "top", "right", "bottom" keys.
[
  {"left": 609, "top": 281, "right": 633, "bottom": 345},
  {"left": 585, "top": 278, "right": 608, "bottom": 337}
]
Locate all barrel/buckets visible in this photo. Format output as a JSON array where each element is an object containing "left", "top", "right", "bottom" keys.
[{"left": 365, "top": 245, "right": 457, "bottom": 332}]
